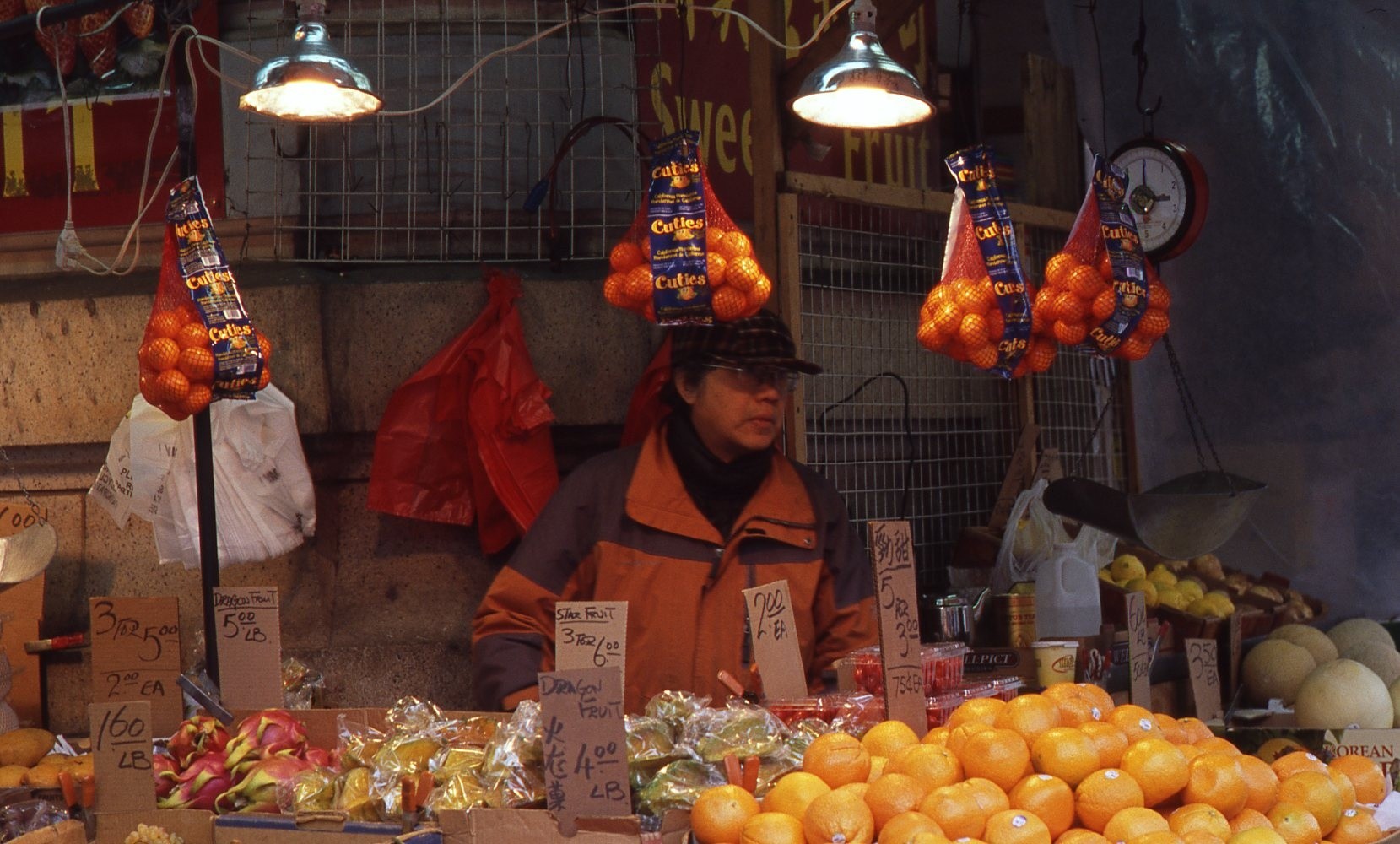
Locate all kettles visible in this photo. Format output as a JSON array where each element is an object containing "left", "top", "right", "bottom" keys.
[{"left": 1032, "top": 641, "right": 1079, "bottom": 691}]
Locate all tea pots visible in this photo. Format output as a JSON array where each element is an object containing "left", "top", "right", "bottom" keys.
[{"left": 918, "top": 588, "right": 991, "bottom": 650}]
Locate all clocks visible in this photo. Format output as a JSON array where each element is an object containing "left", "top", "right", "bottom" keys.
[{"left": 1106, "top": 142, "right": 1212, "bottom": 263}]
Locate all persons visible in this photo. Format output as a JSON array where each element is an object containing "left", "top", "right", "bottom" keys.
[{"left": 469, "top": 304, "right": 882, "bottom": 714}]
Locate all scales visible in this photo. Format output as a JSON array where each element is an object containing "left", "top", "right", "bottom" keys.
[{"left": 1042, "top": 136, "right": 1266, "bottom": 561}]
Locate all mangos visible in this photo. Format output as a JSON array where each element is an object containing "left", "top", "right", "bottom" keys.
[{"left": 1, "top": 722, "right": 97, "bottom": 790}]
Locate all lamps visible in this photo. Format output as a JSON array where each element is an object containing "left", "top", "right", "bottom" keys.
[
  {"left": 787, "top": 0, "right": 942, "bottom": 133},
  {"left": 233, "top": 22, "right": 387, "bottom": 132}
]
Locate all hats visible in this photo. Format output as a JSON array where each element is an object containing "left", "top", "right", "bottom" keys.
[{"left": 670, "top": 308, "right": 822, "bottom": 373}]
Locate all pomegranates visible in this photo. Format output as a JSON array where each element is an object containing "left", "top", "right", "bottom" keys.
[{"left": 1097, "top": 549, "right": 1312, "bottom": 631}]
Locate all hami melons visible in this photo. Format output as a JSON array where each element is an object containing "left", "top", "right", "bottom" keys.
[{"left": 1240, "top": 614, "right": 1399, "bottom": 730}]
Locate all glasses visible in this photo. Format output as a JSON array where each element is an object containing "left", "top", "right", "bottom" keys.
[{"left": 705, "top": 361, "right": 802, "bottom": 391}]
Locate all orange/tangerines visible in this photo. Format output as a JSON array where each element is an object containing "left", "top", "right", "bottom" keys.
[
  {"left": 136, "top": 304, "right": 272, "bottom": 424},
  {"left": 601, "top": 219, "right": 770, "bottom": 324},
  {"left": 915, "top": 233, "right": 1172, "bottom": 382},
  {"left": 671, "top": 677, "right": 1387, "bottom": 840}
]
